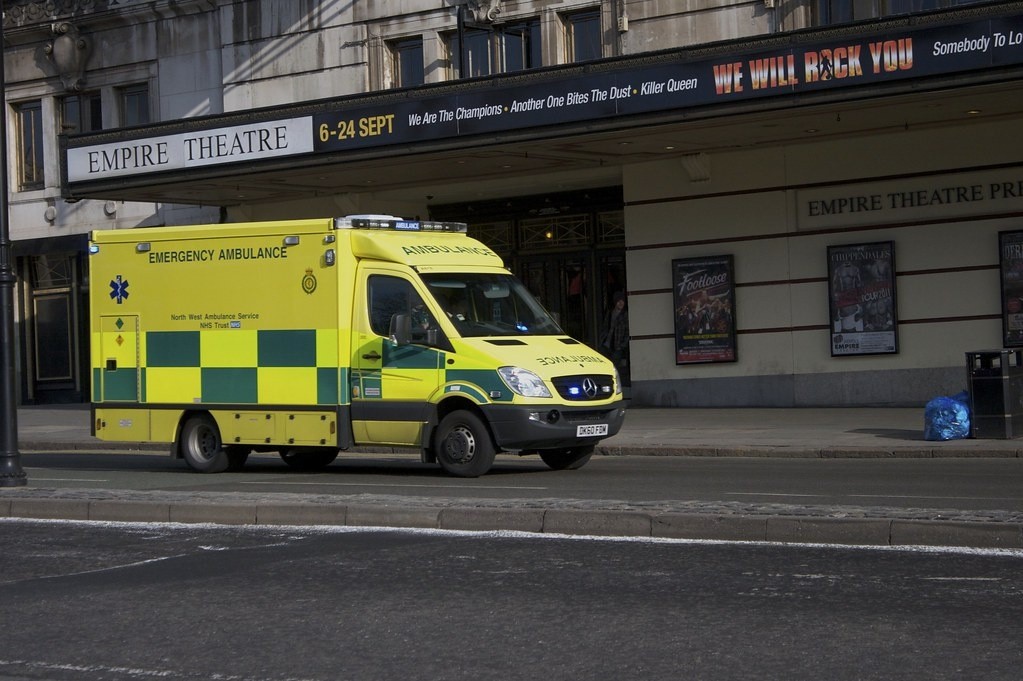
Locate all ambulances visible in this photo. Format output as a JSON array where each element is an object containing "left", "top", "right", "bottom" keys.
[{"left": 89, "top": 214, "right": 627, "bottom": 477}]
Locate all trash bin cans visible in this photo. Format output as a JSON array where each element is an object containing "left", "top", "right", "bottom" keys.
[{"left": 964, "top": 347, "right": 1023, "bottom": 440}]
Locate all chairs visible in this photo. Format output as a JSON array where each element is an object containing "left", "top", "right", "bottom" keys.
[{"left": 390, "top": 313, "right": 412, "bottom": 342}]
